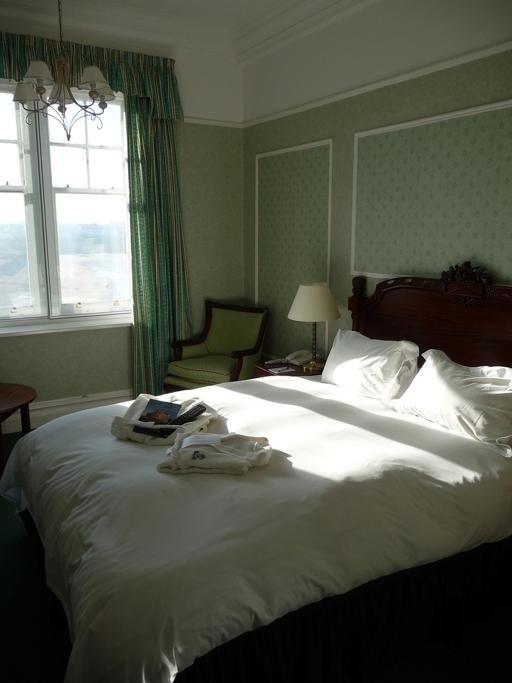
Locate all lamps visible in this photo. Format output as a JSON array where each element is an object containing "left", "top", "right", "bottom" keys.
[
  {"left": 287, "top": 280, "right": 341, "bottom": 371},
  {"left": 12, "top": 0, "right": 117, "bottom": 141}
]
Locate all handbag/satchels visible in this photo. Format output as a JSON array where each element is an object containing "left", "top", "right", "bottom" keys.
[{"left": 110, "top": 393, "right": 219, "bottom": 445}]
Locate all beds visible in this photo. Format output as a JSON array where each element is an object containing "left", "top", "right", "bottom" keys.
[{"left": 16, "top": 258, "right": 511, "bottom": 682}]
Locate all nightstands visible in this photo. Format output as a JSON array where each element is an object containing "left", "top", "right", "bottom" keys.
[{"left": 256, "top": 358, "right": 324, "bottom": 377}]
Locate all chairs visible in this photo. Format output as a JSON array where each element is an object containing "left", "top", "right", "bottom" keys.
[{"left": 160, "top": 299, "right": 269, "bottom": 392}]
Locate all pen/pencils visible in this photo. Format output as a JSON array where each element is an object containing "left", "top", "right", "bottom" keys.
[{"left": 278, "top": 366, "right": 289, "bottom": 371}]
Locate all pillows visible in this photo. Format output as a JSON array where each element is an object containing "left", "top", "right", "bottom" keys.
[{"left": 320, "top": 328, "right": 511, "bottom": 458}]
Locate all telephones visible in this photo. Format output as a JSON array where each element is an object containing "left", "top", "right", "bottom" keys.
[{"left": 286, "top": 349, "right": 314, "bottom": 365}]
